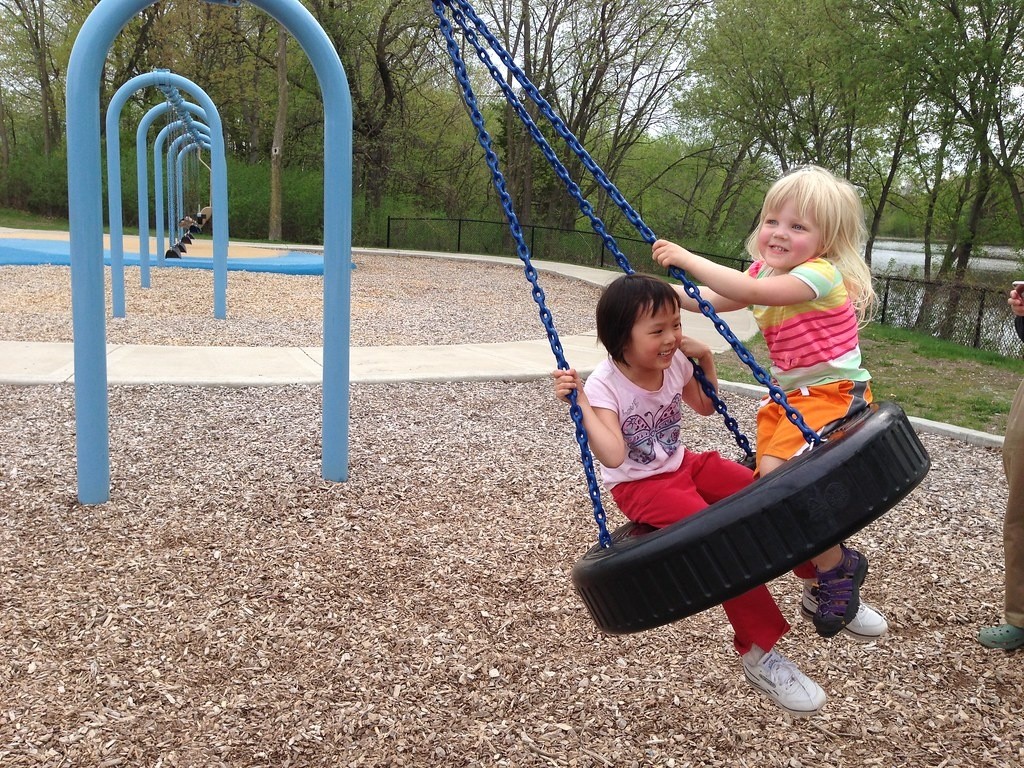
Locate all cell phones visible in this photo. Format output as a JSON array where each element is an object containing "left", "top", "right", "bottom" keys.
[{"left": 1012, "top": 281, "right": 1024, "bottom": 304}]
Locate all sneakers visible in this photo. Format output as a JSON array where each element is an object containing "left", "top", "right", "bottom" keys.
[
  {"left": 799, "top": 581, "right": 888, "bottom": 640},
  {"left": 743, "top": 647, "right": 826, "bottom": 716}
]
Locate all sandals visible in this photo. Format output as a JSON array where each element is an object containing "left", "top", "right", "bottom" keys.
[
  {"left": 977, "top": 623, "right": 1024, "bottom": 650},
  {"left": 813, "top": 542, "right": 869, "bottom": 638}
]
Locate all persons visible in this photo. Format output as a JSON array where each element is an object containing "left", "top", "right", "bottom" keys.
[
  {"left": 652, "top": 165, "right": 880, "bottom": 638},
  {"left": 976, "top": 280, "right": 1024, "bottom": 651},
  {"left": 179, "top": 216, "right": 199, "bottom": 230},
  {"left": 553, "top": 273, "right": 888, "bottom": 717}
]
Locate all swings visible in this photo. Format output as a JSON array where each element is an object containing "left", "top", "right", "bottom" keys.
[
  {"left": 430, "top": 0, "right": 940, "bottom": 638},
  {"left": 151, "top": 66, "right": 205, "bottom": 258}
]
